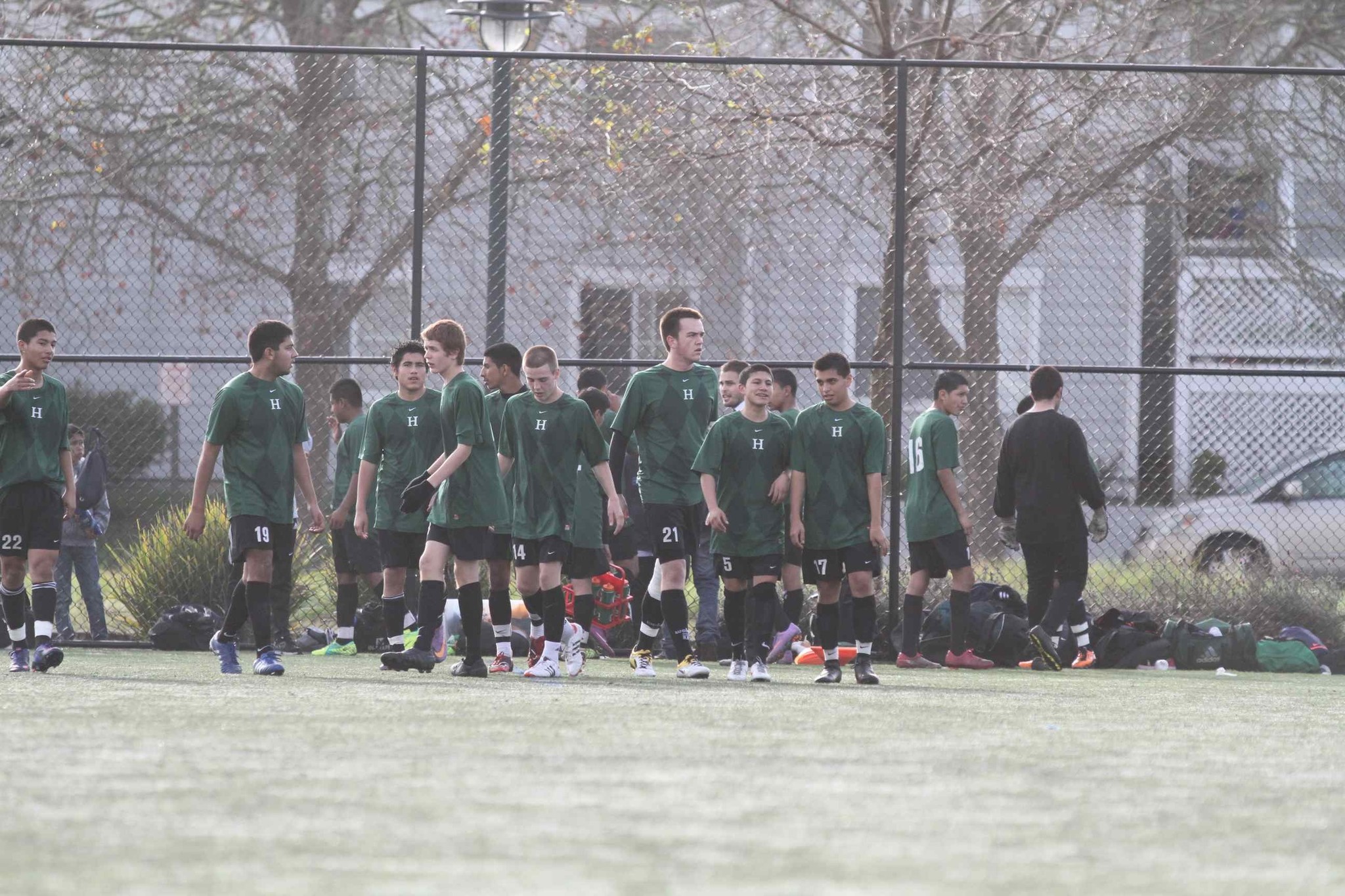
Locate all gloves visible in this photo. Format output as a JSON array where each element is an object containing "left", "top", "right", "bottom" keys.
[
  {"left": 1088, "top": 507, "right": 1110, "bottom": 543},
  {"left": 400, "top": 480, "right": 436, "bottom": 516},
  {"left": 400, "top": 471, "right": 431, "bottom": 495},
  {"left": 999, "top": 516, "right": 1021, "bottom": 551}
]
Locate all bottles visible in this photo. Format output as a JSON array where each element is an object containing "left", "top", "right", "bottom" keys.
[{"left": 588, "top": 570, "right": 631, "bottom": 624}]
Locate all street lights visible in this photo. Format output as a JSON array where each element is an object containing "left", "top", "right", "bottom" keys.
[{"left": 441, "top": 0, "right": 565, "bottom": 367}]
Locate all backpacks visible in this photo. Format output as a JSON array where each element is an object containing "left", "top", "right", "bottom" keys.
[
  {"left": 61, "top": 428, "right": 108, "bottom": 514},
  {"left": 143, "top": 583, "right": 1332, "bottom": 689}
]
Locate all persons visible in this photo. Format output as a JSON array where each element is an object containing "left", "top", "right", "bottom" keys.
[
  {"left": 57, "top": 422, "right": 112, "bottom": 639},
  {"left": 2, "top": 317, "right": 79, "bottom": 674},
  {"left": 176, "top": 305, "right": 1114, "bottom": 683}
]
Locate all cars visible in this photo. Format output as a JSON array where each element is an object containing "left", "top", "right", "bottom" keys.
[{"left": 1120, "top": 444, "right": 1344, "bottom": 592}]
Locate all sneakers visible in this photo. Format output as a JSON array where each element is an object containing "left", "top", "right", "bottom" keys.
[{"left": 6, "top": 616, "right": 1112, "bottom": 713}]
